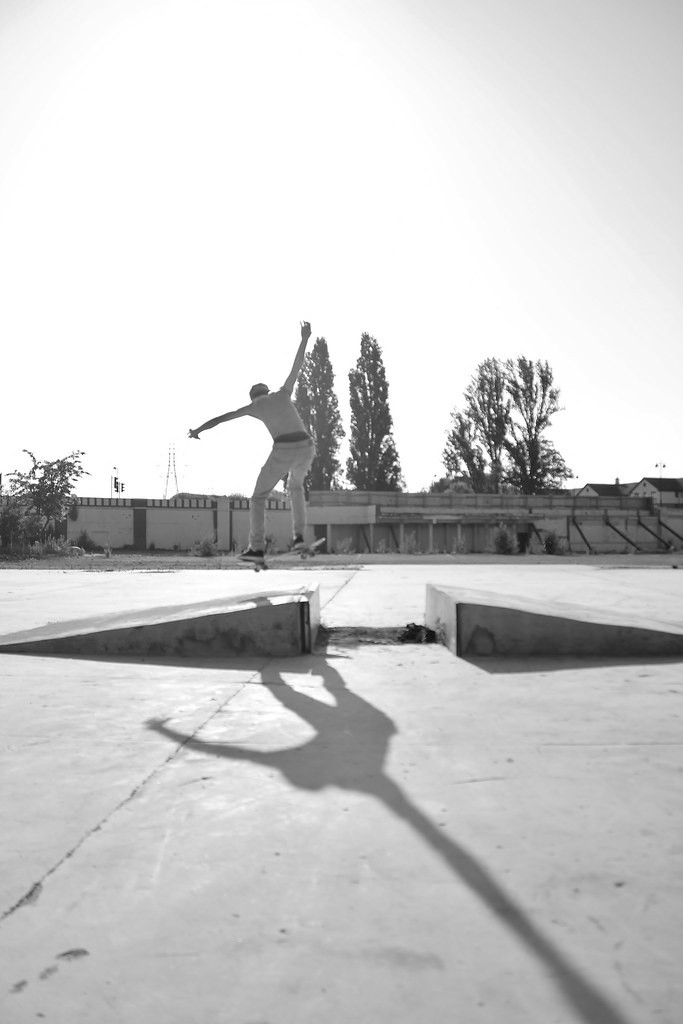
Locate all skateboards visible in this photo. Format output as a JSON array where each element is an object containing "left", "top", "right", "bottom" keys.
[{"left": 233, "top": 536, "right": 326, "bottom": 572}]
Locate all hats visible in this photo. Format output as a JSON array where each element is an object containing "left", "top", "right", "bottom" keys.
[{"left": 249, "top": 383, "right": 270, "bottom": 398}]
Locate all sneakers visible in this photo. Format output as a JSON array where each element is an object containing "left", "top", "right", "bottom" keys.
[
  {"left": 237, "top": 548, "right": 264, "bottom": 562},
  {"left": 290, "top": 536, "right": 305, "bottom": 550}
]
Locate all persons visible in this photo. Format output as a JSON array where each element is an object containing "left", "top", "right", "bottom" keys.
[{"left": 193, "top": 320, "right": 315, "bottom": 562}]
